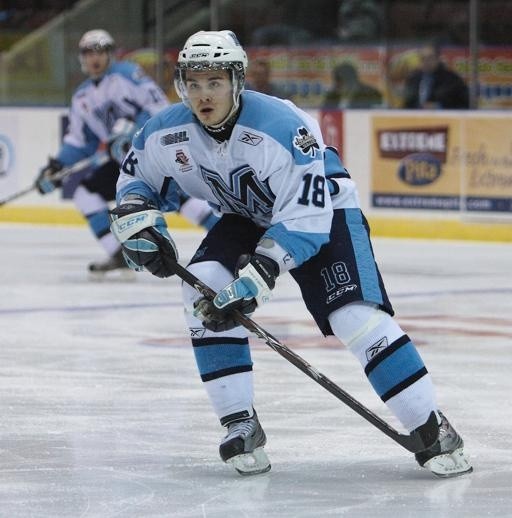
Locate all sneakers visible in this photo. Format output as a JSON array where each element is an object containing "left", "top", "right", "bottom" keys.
[
  {"left": 416, "top": 410, "right": 463, "bottom": 466},
  {"left": 219, "top": 408, "right": 266, "bottom": 462},
  {"left": 89, "top": 250, "right": 129, "bottom": 271}
]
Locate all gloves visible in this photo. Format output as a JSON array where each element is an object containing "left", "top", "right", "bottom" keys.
[
  {"left": 194, "top": 253, "right": 279, "bottom": 332},
  {"left": 107, "top": 203, "right": 179, "bottom": 278},
  {"left": 36, "top": 158, "right": 64, "bottom": 193},
  {"left": 106, "top": 135, "right": 132, "bottom": 164}
]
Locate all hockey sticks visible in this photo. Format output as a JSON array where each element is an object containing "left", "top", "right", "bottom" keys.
[{"left": 160, "top": 251, "right": 438, "bottom": 453}]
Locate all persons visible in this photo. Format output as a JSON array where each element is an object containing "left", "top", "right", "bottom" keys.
[
  {"left": 243, "top": 59, "right": 296, "bottom": 106},
  {"left": 30, "top": 26, "right": 172, "bottom": 276},
  {"left": 108, "top": 24, "right": 466, "bottom": 468},
  {"left": 399, "top": 39, "right": 472, "bottom": 111},
  {"left": 320, "top": 61, "right": 384, "bottom": 112}
]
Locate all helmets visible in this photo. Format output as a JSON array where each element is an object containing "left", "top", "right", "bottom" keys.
[
  {"left": 174, "top": 31, "right": 247, "bottom": 106},
  {"left": 80, "top": 30, "right": 113, "bottom": 53}
]
